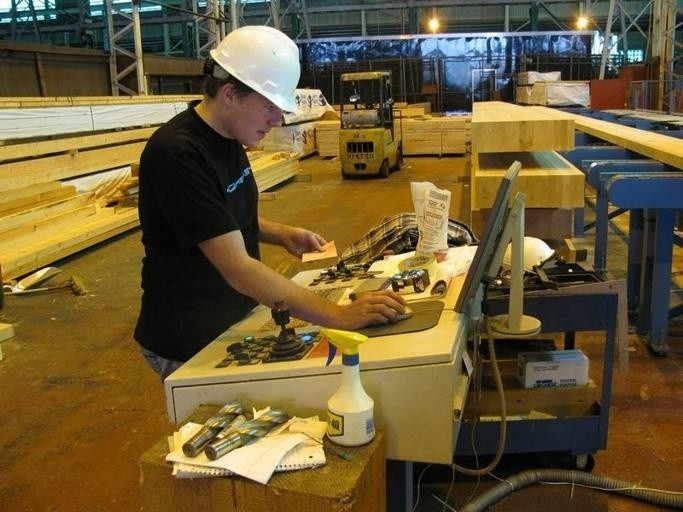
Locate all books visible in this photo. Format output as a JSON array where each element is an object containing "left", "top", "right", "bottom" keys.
[{"left": 168, "top": 415, "right": 326, "bottom": 481}]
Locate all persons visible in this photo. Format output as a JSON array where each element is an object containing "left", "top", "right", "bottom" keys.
[{"left": 133, "top": 25, "right": 405, "bottom": 381}]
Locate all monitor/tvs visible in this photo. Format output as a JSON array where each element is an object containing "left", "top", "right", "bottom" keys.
[{"left": 453, "top": 161, "right": 541, "bottom": 338}]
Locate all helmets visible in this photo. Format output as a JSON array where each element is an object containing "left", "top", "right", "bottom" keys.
[
  {"left": 207, "top": 25, "right": 301, "bottom": 114},
  {"left": 503, "top": 236, "right": 556, "bottom": 279}
]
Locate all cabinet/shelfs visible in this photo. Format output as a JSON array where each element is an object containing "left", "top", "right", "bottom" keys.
[
  {"left": 452, "top": 239, "right": 618, "bottom": 475},
  {"left": 296, "top": 54, "right": 649, "bottom": 117},
  {"left": 136, "top": 402, "right": 390, "bottom": 512}
]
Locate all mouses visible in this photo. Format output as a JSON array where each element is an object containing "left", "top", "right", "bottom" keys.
[{"left": 391, "top": 307, "right": 412, "bottom": 321}]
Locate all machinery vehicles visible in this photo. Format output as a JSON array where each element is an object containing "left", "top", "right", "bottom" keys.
[
  {"left": 338, "top": 71, "right": 403, "bottom": 179},
  {"left": 418, "top": 66, "right": 443, "bottom": 109}
]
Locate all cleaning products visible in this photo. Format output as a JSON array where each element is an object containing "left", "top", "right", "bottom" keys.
[{"left": 318, "top": 327, "right": 377, "bottom": 449}]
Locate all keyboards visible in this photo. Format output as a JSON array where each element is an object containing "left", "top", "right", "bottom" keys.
[{"left": 261, "top": 286, "right": 347, "bottom": 331}]
